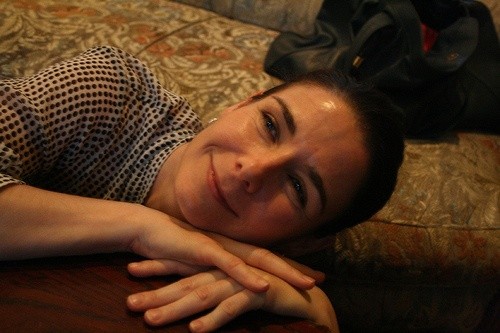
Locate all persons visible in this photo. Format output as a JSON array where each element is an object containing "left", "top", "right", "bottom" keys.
[{"left": 0, "top": 44, "right": 406, "bottom": 333}]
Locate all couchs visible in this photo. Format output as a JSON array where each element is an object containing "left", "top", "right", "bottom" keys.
[{"left": 1, "top": 1, "right": 498, "bottom": 286}]
